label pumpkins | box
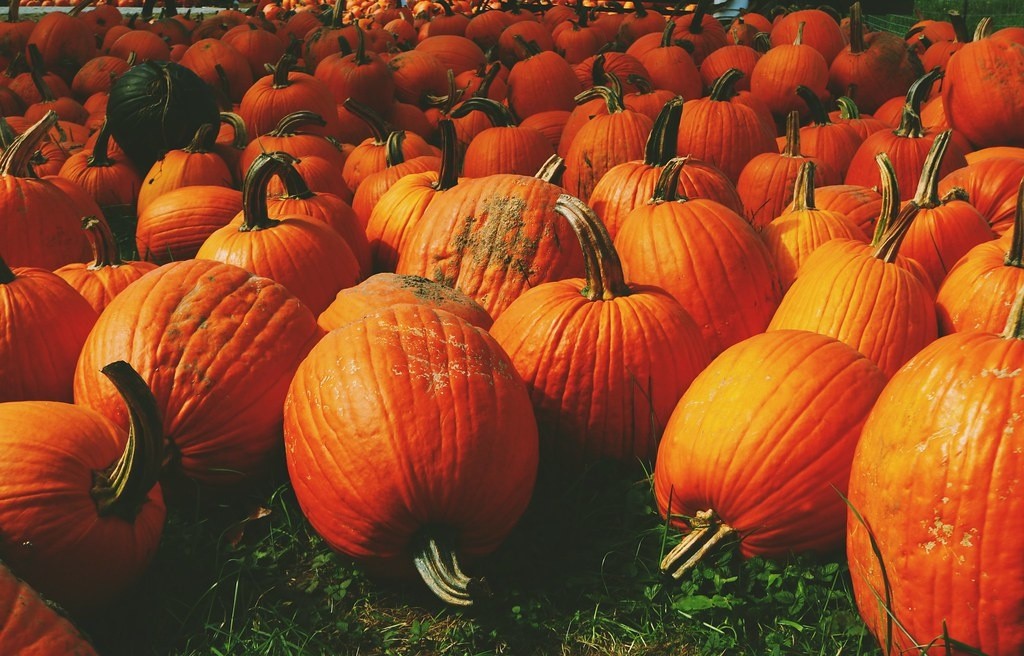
[0,0,1024,656]
